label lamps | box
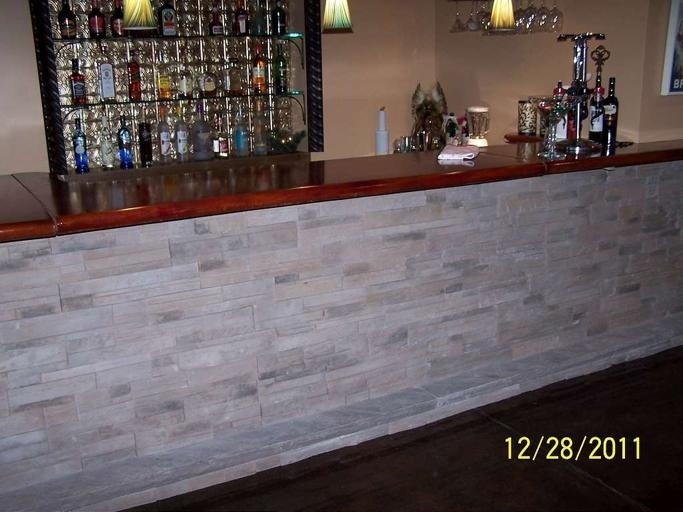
[121,0,158,31]
[321,0,354,34]
[489,0,516,31]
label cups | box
[465,105,491,146]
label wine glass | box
[526,96,579,160]
[449,0,563,33]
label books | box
[436,144,480,160]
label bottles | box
[554,80,565,99]
[58,1,291,38]
[71,108,292,171]
[67,36,288,107]
[589,75,618,146]
[375,106,389,156]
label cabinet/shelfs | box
[29,0,324,172]
